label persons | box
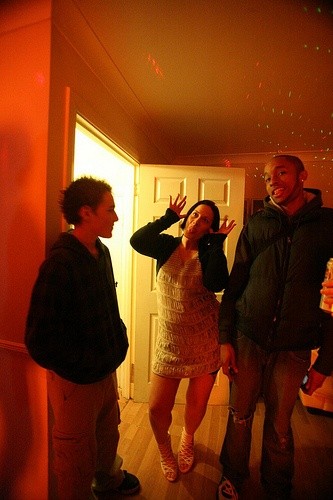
[320,280,333,305]
[24,175,140,500]
[216,155,333,500]
[130,193,237,482]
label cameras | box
[300,373,309,390]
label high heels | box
[177,426,195,473]
[157,431,178,482]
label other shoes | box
[92,470,140,495]
[217,475,239,500]
